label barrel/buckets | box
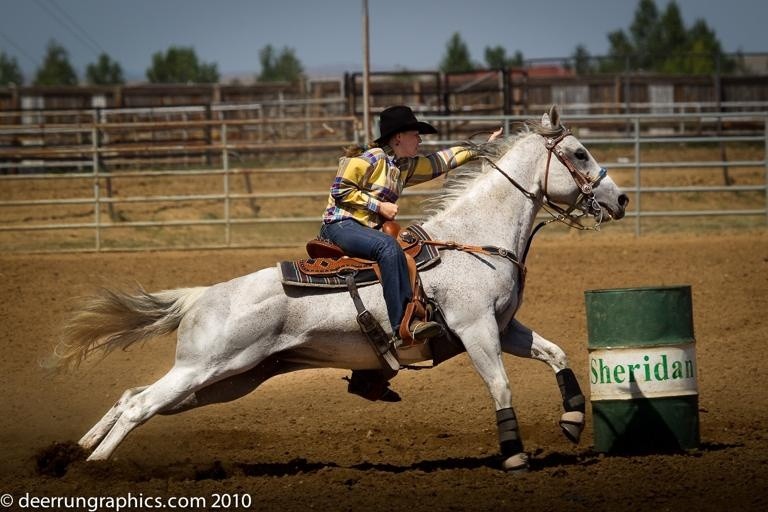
[584,285,700,454]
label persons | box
[320,127,503,350]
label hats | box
[374,106,437,143]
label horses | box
[35,103,630,476]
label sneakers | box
[394,316,442,351]
